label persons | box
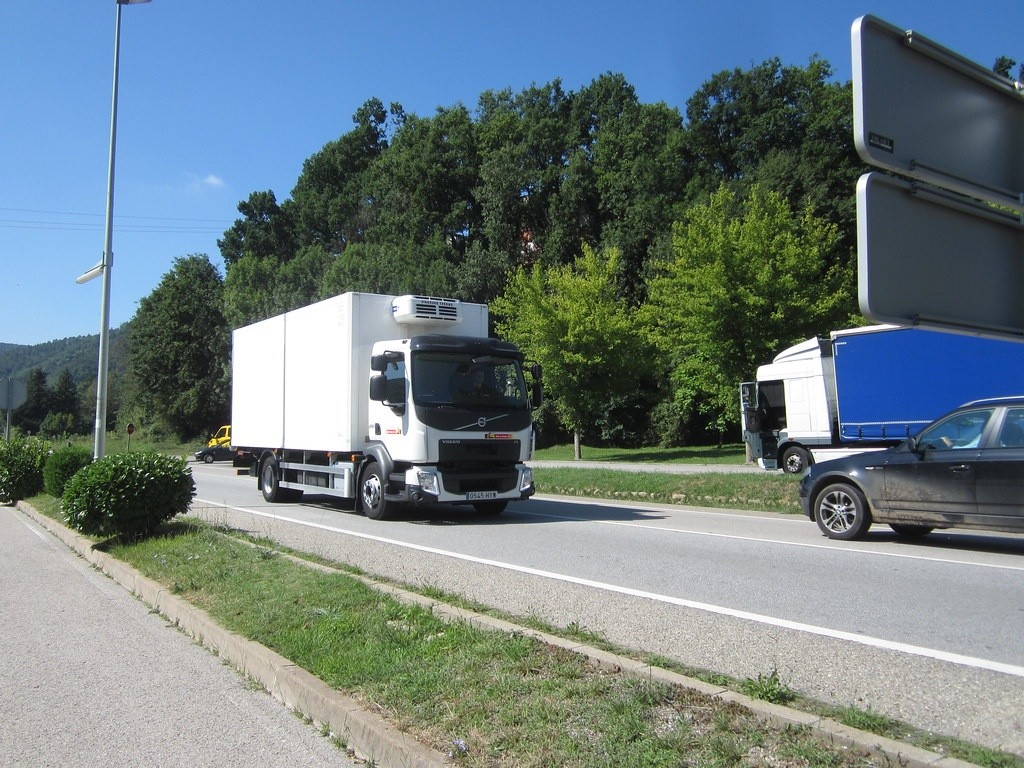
[461,369,502,397]
[940,419,1006,449]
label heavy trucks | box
[739,314,1024,475]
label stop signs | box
[127,424,134,435]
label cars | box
[195,439,234,463]
[799,391,1024,540]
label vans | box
[208,425,231,448]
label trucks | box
[230,289,544,519]
